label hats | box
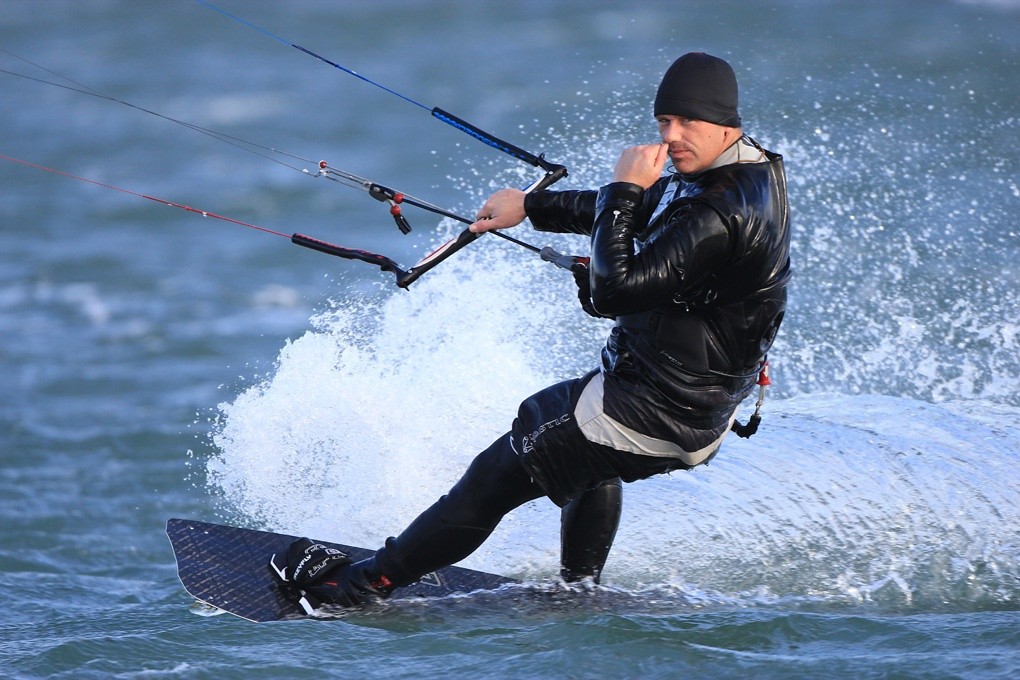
[654,51,742,128]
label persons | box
[288,52,793,609]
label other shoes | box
[287,538,389,617]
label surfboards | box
[165,516,526,627]
